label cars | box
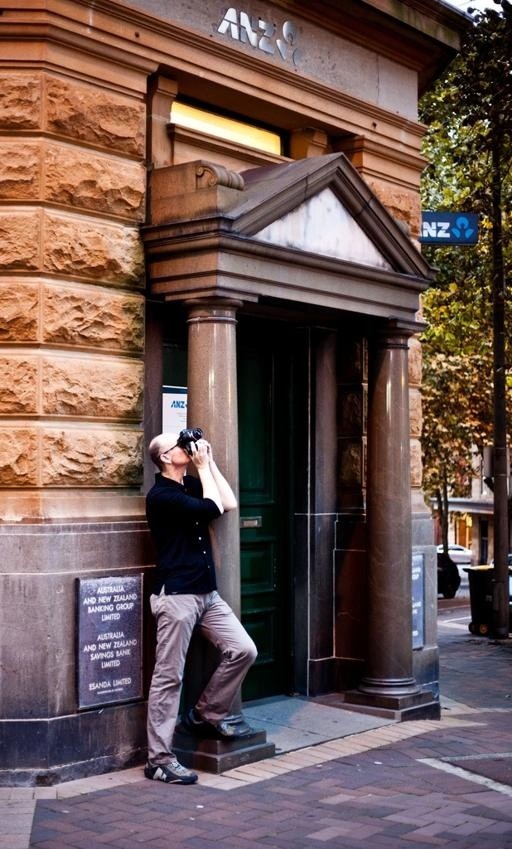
[435,544,472,565]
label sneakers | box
[189,708,253,739]
[143,758,199,786]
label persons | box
[141,430,259,786]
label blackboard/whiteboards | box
[74,573,144,710]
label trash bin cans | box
[462,564,512,639]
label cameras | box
[178,427,204,454]
